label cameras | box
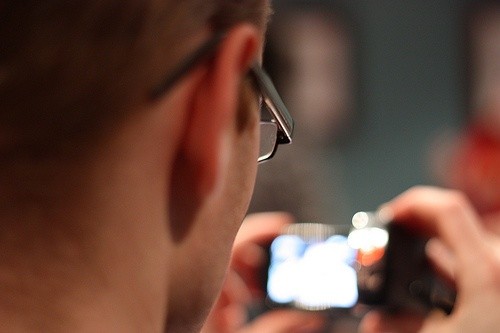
[264,221,457,316]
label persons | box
[0,0,500,332]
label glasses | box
[149,39,293,161]
